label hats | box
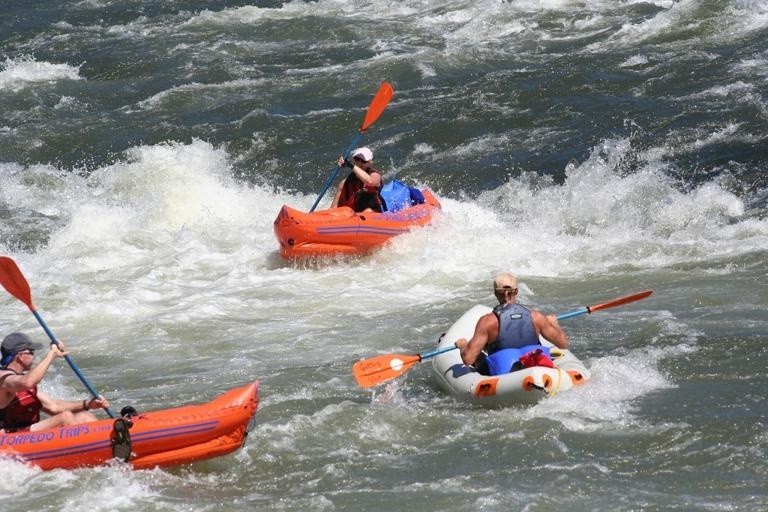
[352,148,373,161]
[494,273,517,292]
[1,333,44,356]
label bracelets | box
[83,400,88,410]
[348,161,355,169]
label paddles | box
[309,82,395,213]
[352,289,652,388]
[0,256,117,418]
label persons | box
[0,333,109,435]
[455,275,569,375]
[331,147,384,213]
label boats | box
[430,303,592,407]
[271,183,443,264]
[0,379,263,472]
